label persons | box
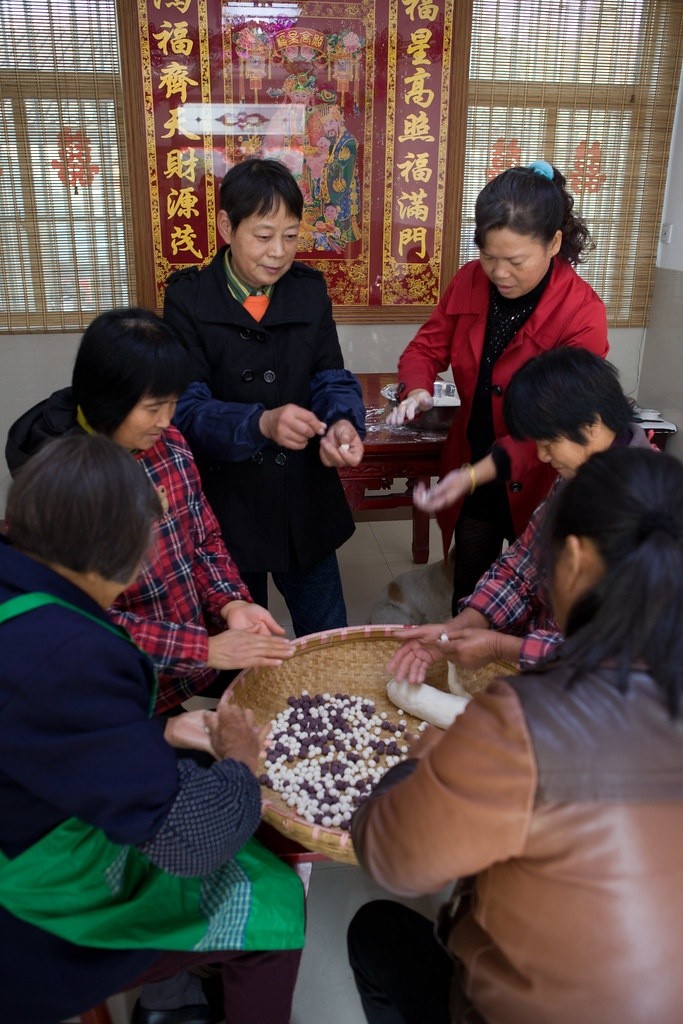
[385,161,611,618]
[0,432,308,1024]
[347,446,683,1024]
[163,159,366,641]
[5,307,337,862]
[383,334,662,687]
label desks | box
[334,372,460,564]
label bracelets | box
[461,463,478,500]
